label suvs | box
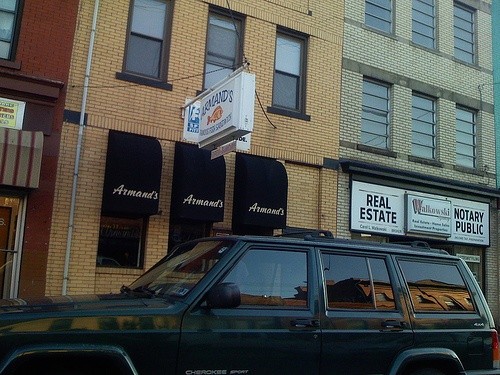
[0,229,500,375]
